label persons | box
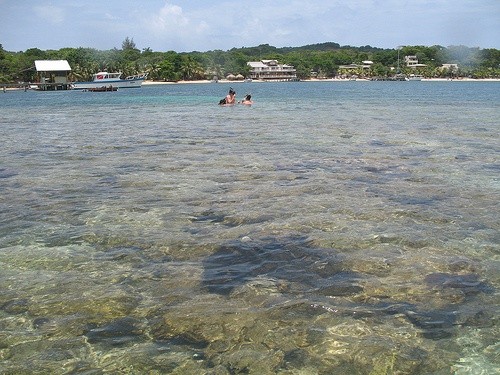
[218,98,226,106]
[226,90,237,105]
[240,95,254,104]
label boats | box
[63,72,149,88]
